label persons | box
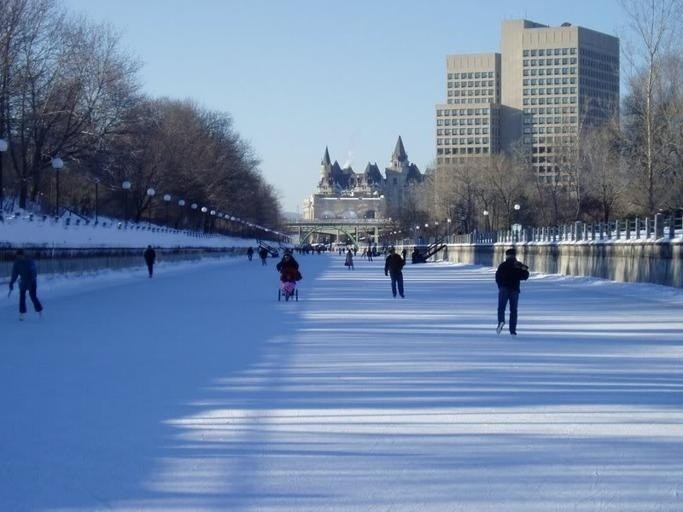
[244,242,419,298]
[9,250,42,322]
[494,247,530,336]
[143,245,155,278]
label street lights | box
[146,188,155,226]
[50,157,62,218]
[0,138,9,218]
[120,180,132,228]
[163,193,171,227]
[446,218,451,243]
[482,210,489,243]
[380,219,439,244]
[178,198,291,244]
[513,203,521,242]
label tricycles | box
[277,269,299,303]
